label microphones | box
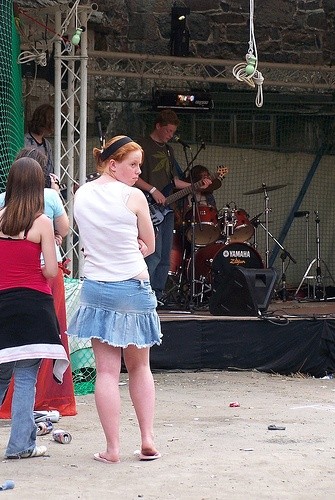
[174,135,190,148]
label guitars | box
[146,164,228,225]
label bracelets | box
[149,187,156,194]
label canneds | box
[229,402,240,407]
[34,410,60,435]
[52,430,72,443]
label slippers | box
[93,452,120,464]
[140,450,161,460]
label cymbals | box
[249,212,263,223]
[244,185,286,195]
[252,220,274,225]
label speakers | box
[209,265,280,316]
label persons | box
[0,158,68,458]
[0,147,77,418]
[21,104,53,157]
[130,109,212,308]
[180,165,216,215]
[64,136,163,464]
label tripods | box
[157,149,335,313]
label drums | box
[184,203,220,246]
[165,229,188,276]
[185,240,265,297]
[227,208,254,245]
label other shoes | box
[155,297,168,306]
[7,446,48,459]
